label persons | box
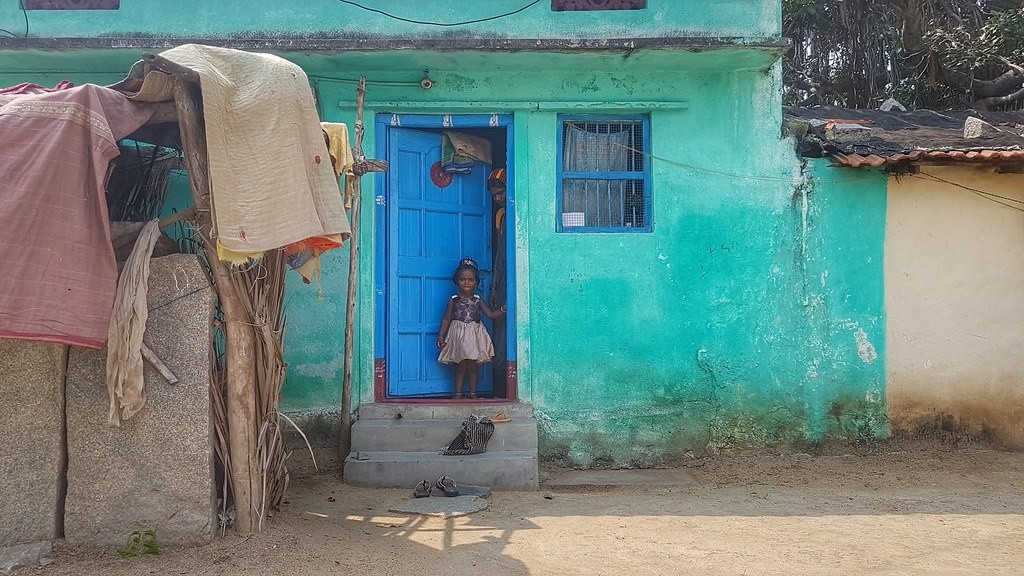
[487,167,506,398]
[438,258,506,399]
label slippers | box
[413,479,432,498]
[436,475,459,496]
[490,412,512,423]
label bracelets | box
[437,337,444,338]
[500,306,506,313]
[490,288,497,290]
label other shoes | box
[452,393,463,399]
[467,393,477,400]
